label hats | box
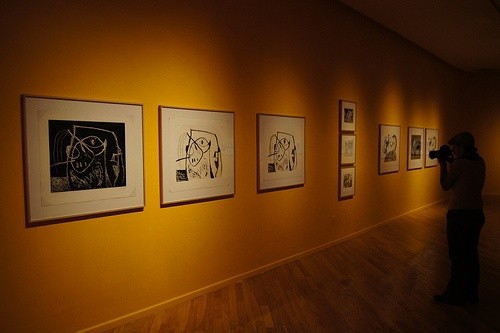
[448,132,475,147]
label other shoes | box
[433,290,480,311]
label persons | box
[433,131,486,310]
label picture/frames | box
[21,94,145,223]
[340,167,355,197]
[256,113,305,194]
[407,127,423,171]
[425,128,439,168]
[158,105,234,208]
[341,134,356,165]
[379,124,400,175]
[340,99,356,133]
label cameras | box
[429,145,454,163]
